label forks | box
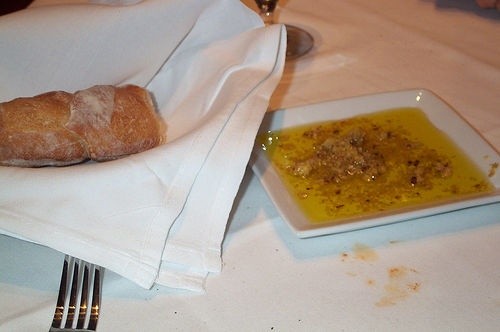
[48,255,102,332]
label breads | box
[0,85,164,167]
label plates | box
[250,88,500,239]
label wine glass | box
[254,0,313,59]
